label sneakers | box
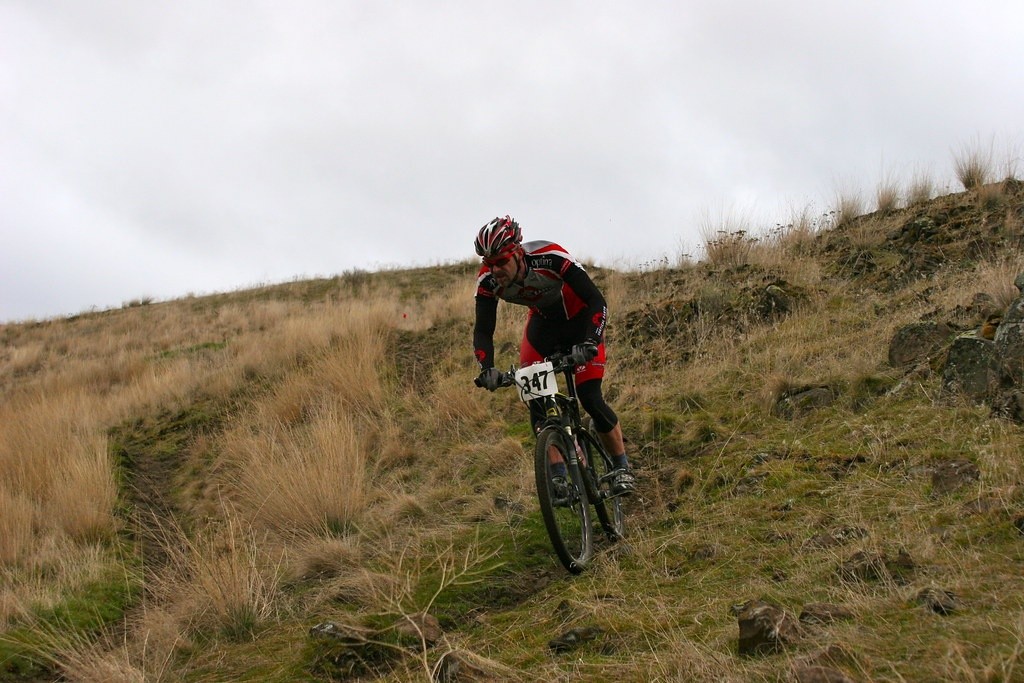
[552,477,572,508]
[610,465,635,497]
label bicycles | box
[474,346,633,575]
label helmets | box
[474,216,522,258]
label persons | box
[472,215,635,506]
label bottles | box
[573,440,587,468]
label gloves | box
[478,369,503,393]
[572,340,598,366]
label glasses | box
[482,248,519,267]
